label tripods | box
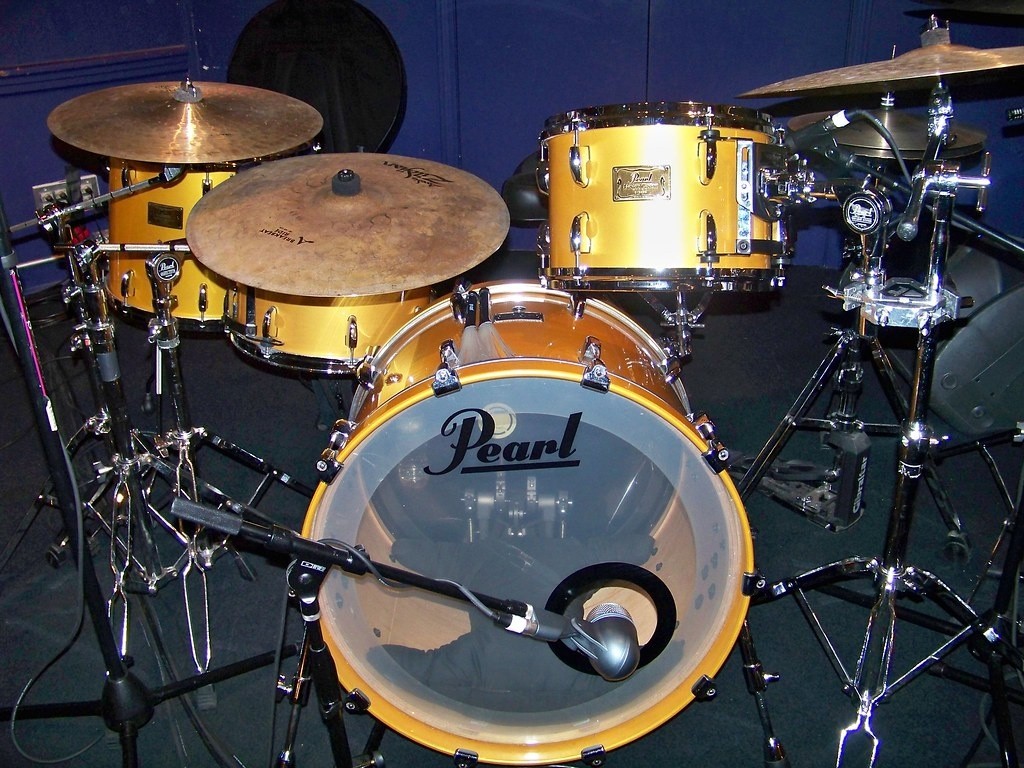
[731,85,1024,768]
[0,163,320,768]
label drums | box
[101,152,234,335]
[291,280,756,768]
[221,281,443,376]
[535,101,802,298]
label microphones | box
[583,603,640,681]
[783,107,859,154]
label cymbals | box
[790,105,988,165]
[738,44,1024,99]
[47,78,327,167]
[183,152,512,298]
